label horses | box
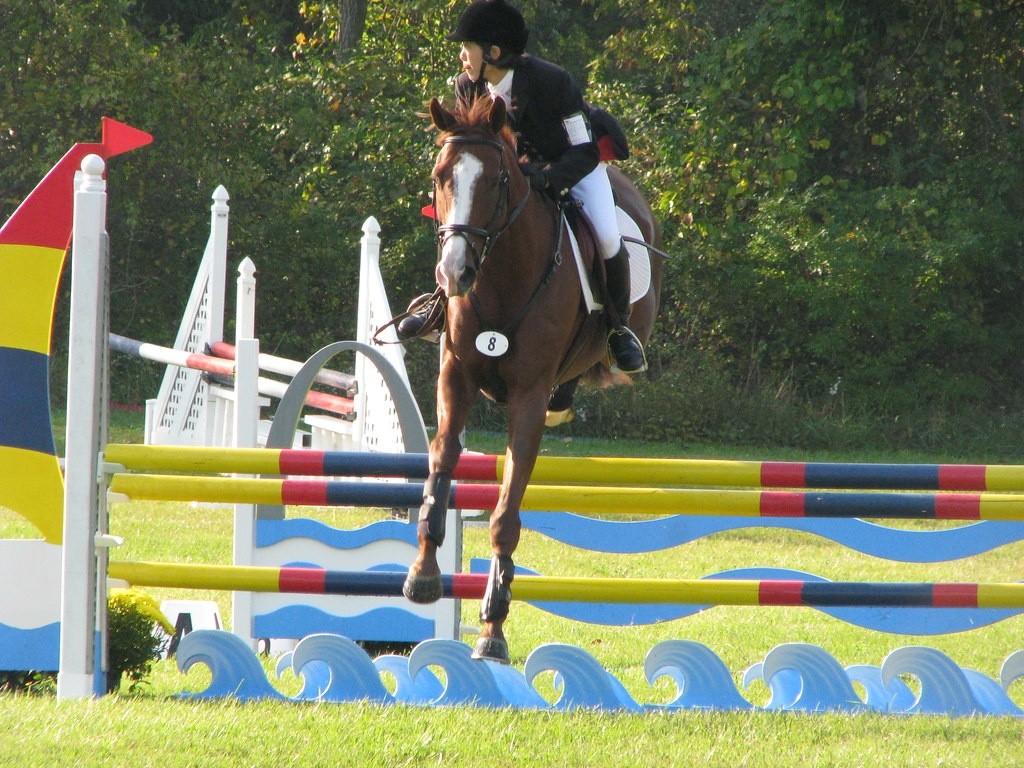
[401,90,664,665]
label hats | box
[444,0,530,52]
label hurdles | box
[0,140,1023,715]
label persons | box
[397,1,644,369]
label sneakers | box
[607,320,646,372]
[398,298,445,335]
